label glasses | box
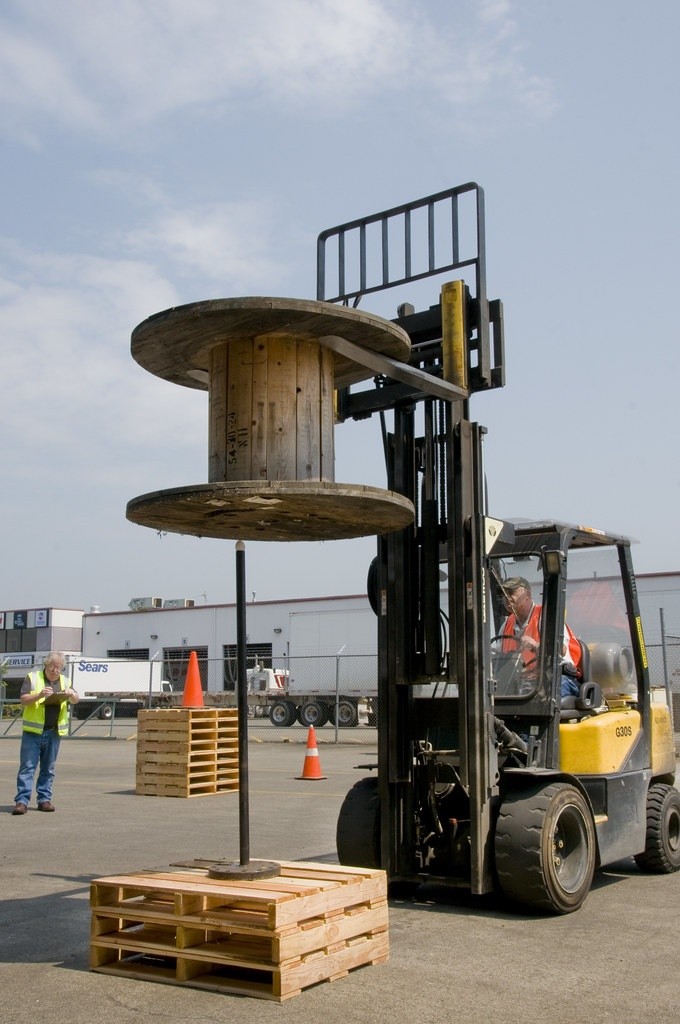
[47,664,63,671]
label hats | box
[496,576,531,591]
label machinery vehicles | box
[188,181,680,916]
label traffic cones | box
[293,725,328,780]
[173,651,210,710]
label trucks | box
[65,655,173,720]
[85,656,378,726]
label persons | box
[490,576,584,697]
[12,651,79,815]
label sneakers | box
[38,801,55,811]
[13,803,28,815]
